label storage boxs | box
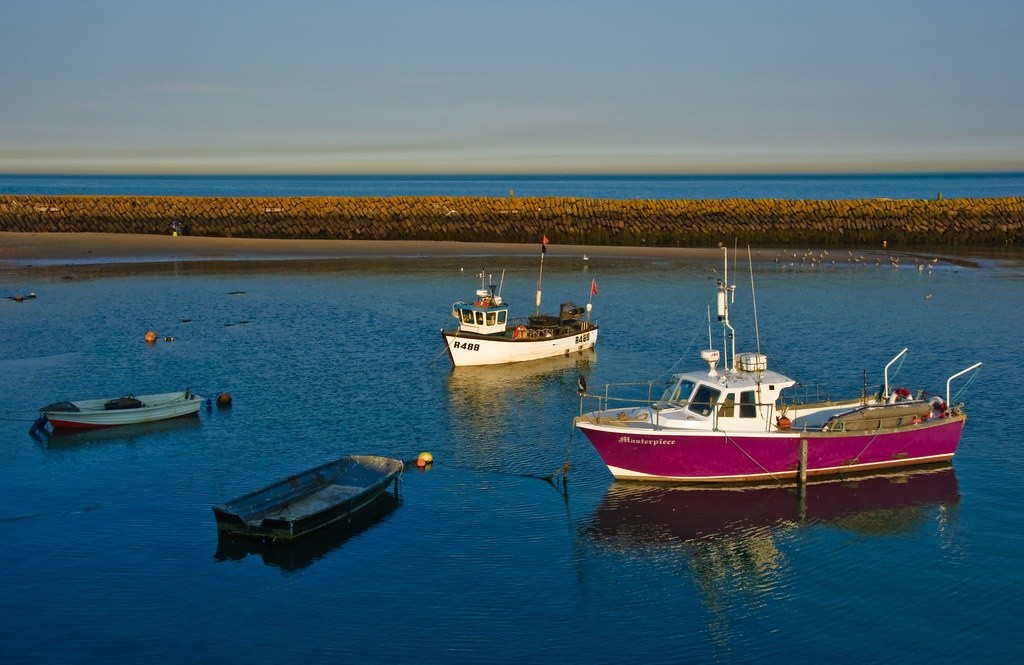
[734,352,769,370]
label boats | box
[28,385,206,436]
[211,456,403,540]
[440,234,599,367]
[573,238,982,484]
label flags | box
[593,282,598,294]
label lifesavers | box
[512,325,528,338]
[887,388,913,405]
[926,396,947,421]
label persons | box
[690,388,702,414]
